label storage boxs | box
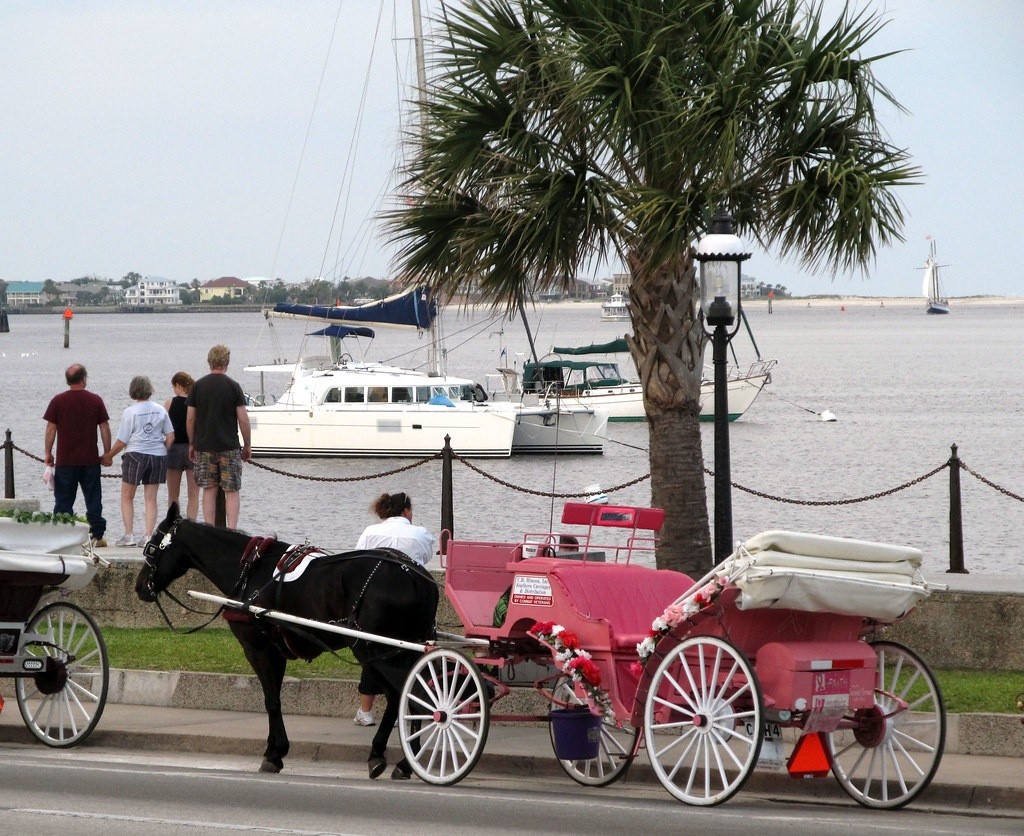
[755,639,878,712]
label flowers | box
[634,574,730,662]
[529,621,615,721]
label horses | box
[135,500,440,781]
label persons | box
[185,344,251,530]
[42,363,113,547]
[165,372,196,523]
[544,533,582,555]
[353,492,433,726]
[99,375,175,546]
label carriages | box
[136,385,947,810]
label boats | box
[600,292,632,323]
[484,304,778,422]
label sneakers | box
[353,707,375,726]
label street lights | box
[691,202,754,570]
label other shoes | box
[115,533,136,546]
[95,539,107,547]
[138,534,152,548]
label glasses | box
[400,492,408,503]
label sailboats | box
[239,0,608,459]
[914,236,952,315]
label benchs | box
[506,535,663,577]
[551,566,696,649]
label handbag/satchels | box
[493,585,511,627]
